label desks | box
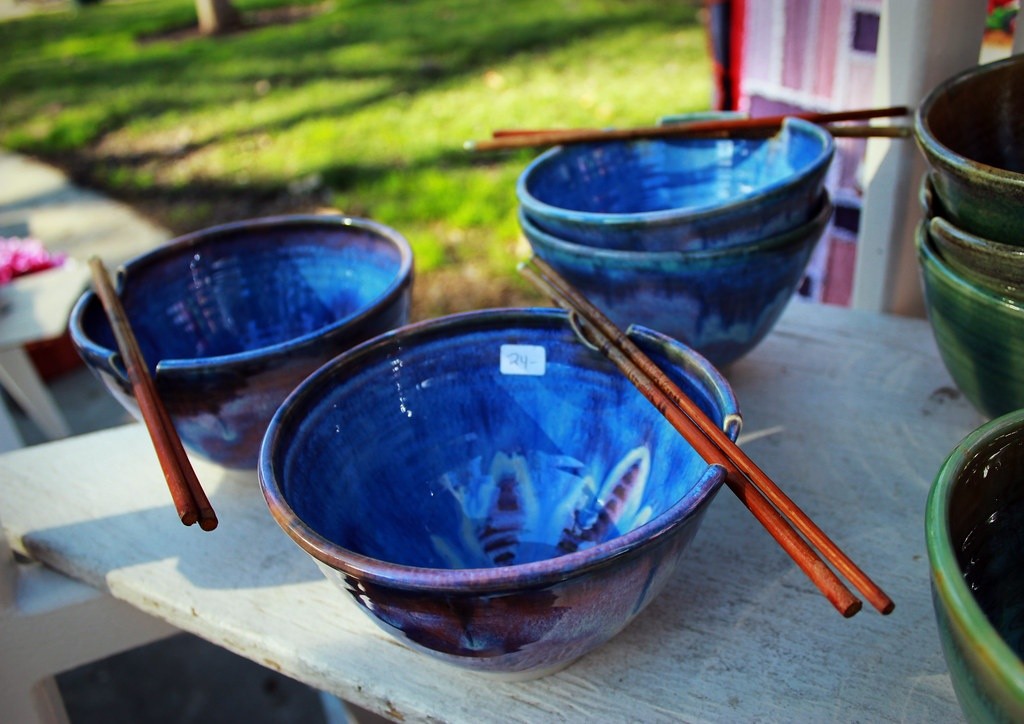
[1,292,994,724]
[1,150,180,440]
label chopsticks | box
[522,256,896,619]
[90,255,219,533]
[465,106,911,153]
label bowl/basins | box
[918,171,1024,298]
[259,307,741,683]
[518,112,835,251]
[70,213,415,471]
[924,410,1024,724]
[520,184,832,375]
[915,218,1024,419]
[913,53,1024,247]
